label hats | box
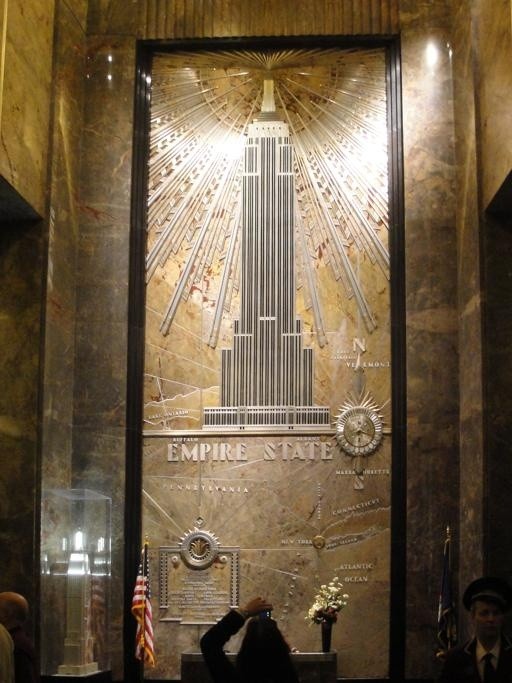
[462,577,512,607]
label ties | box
[480,653,497,683]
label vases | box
[320,621,333,652]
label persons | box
[0,592,29,683]
[438,576,512,683]
[200,597,298,683]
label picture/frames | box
[118,29,409,683]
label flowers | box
[303,576,348,627]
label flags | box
[132,539,155,668]
[86,545,106,664]
[436,538,458,662]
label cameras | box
[259,610,271,620]
